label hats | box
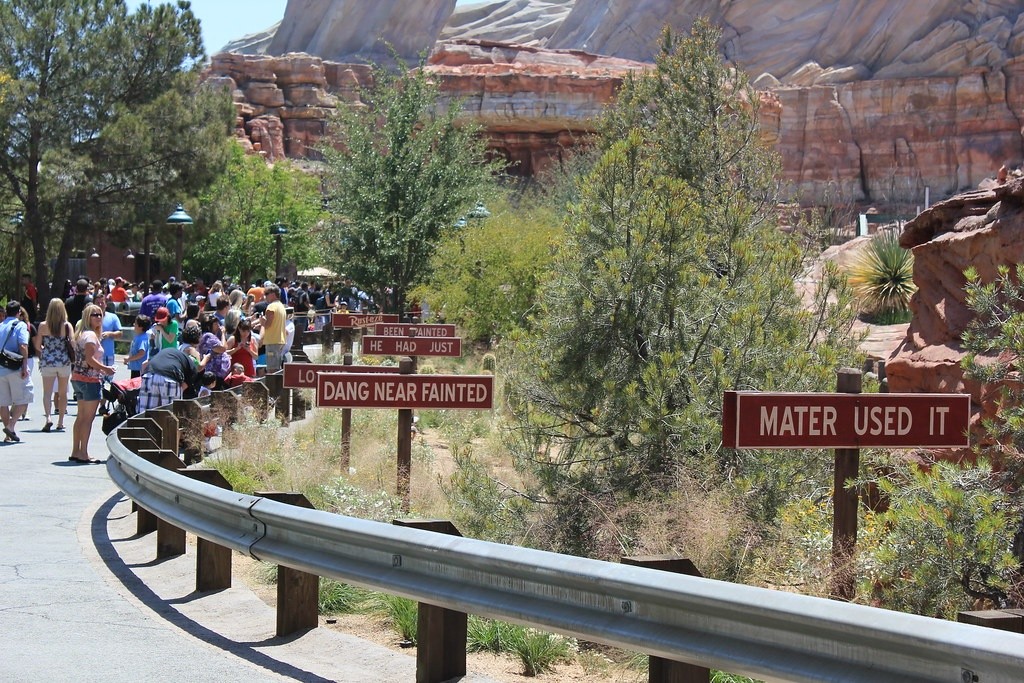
[341,301,347,305]
[155,306,170,322]
[153,280,163,289]
[77,279,88,291]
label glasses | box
[90,314,102,317]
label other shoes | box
[22,415,30,420]
[42,423,53,432]
[55,409,68,414]
[3,428,20,442]
[99,405,106,415]
[68,456,101,464]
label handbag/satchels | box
[65,321,75,363]
[307,309,315,318]
[0,349,24,370]
[102,411,127,436]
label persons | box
[148,307,178,360]
[123,314,152,379]
[196,313,232,392]
[68,305,116,464]
[1,275,435,464]
[260,283,287,374]
[36,298,76,432]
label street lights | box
[166,203,194,284]
[466,200,492,228]
[10,211,28,303]
[453,216,473,255]
[269,226,288,278]
